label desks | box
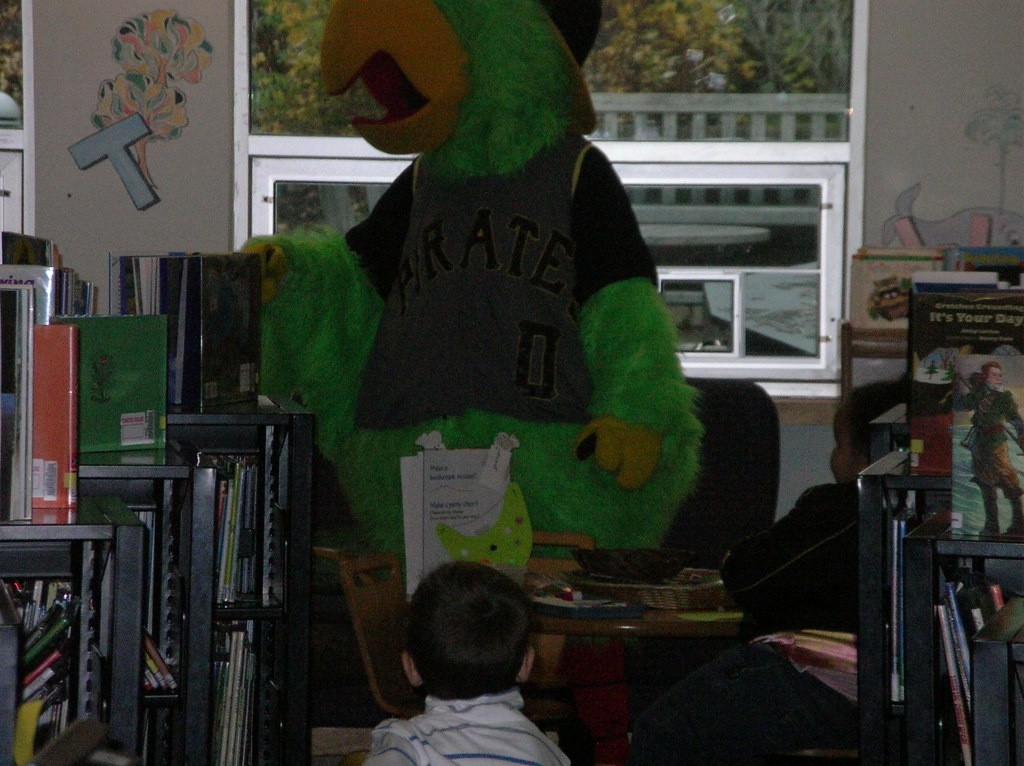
[525,606,741,766]
[640,224,770,266]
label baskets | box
[563,570,724,612]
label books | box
[0,228,277,766]
[846,242,1024,766]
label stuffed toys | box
[237,0,707,552]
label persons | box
[360,562,573,766]
[724,375,911,645]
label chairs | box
[525,532,594,682]
[340,552,426,716]
[658,374,782,550]
